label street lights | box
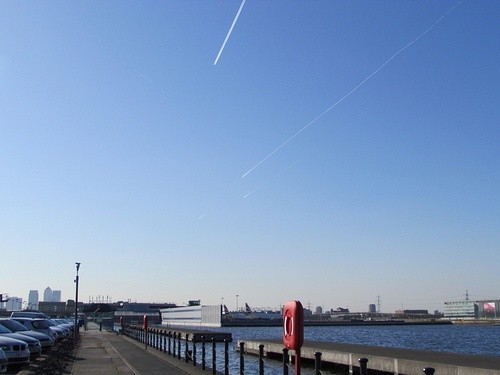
[74,262,81,334]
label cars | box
[0,311,79,373]
